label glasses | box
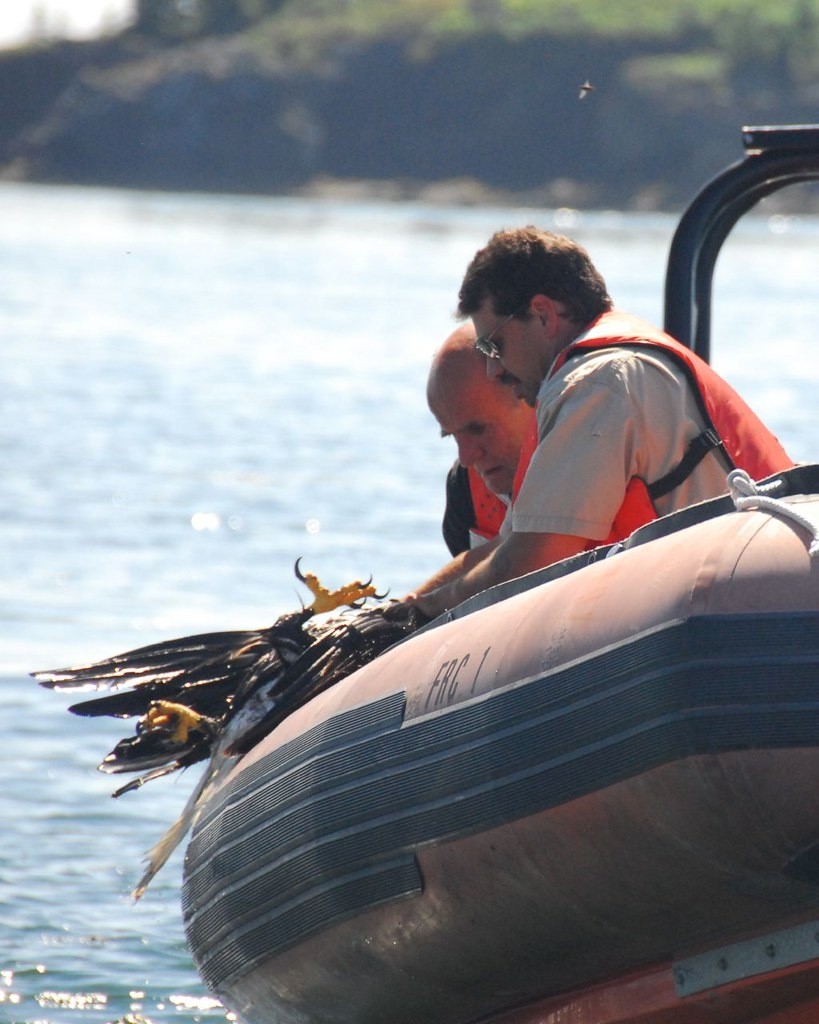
[474,305,529,358]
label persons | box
[404,224,794,620]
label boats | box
[174,122,819,1024]
